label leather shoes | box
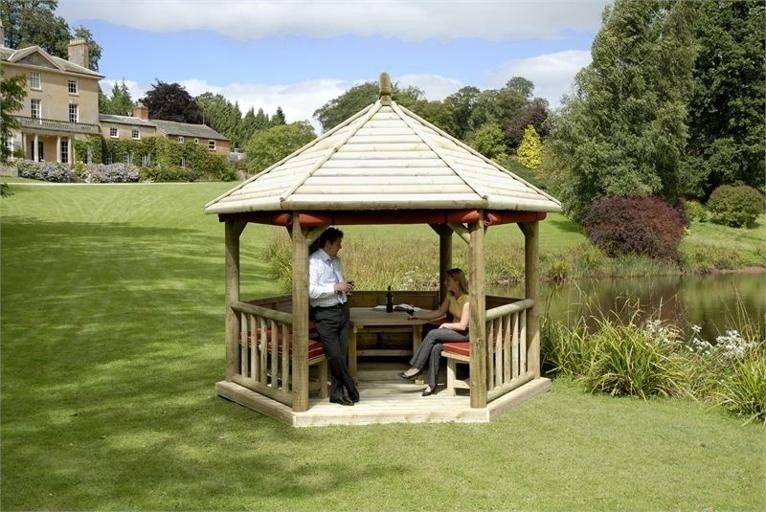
[329,395,353,406]
[343,378,359,402]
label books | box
[371,301,420,312]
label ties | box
[329,260,345,304]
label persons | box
[306,226,360,407]
[398,268,472,396]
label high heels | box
[400,367,422,379]
[422,384,437,396]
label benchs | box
[268,318,321,340]
[426,315,452,329]
[237,328,331,399]
[439,333,517,400]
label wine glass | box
[406,305,415,319]
[347,280,354,295]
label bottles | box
[385,284,394,312]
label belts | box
[315,303,344,310]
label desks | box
[339,307,447,389]
